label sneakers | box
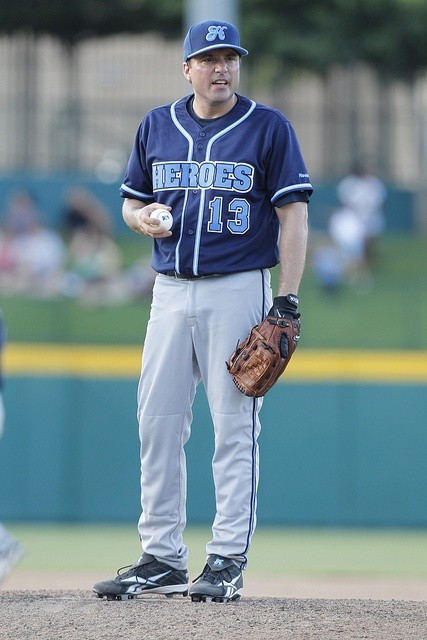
[187,554,244,602]
[92,553,189,603]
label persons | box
[93,20,312,599]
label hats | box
[183,20,249,60]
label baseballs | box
[149,209,174,231]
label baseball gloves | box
[224,293,302,398]
